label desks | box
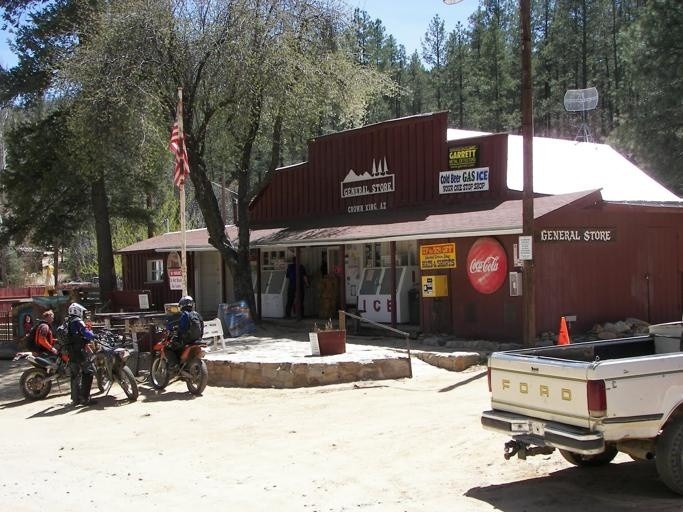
[88,310,168,345]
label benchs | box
[199,317,226,352]
[105,288,157,313]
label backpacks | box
[183,311,202,340]
[26,323,42,352]
[56,317,77,346]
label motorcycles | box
[11,338,96,401]
[93,329,138,401]
[148,323,208,395]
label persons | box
[62,302,99,406]
[169,294,206,371]
[284,255,309,317]
[32,311,66,377]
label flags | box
[165,100,191,191]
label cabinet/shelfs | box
[345,243,384,305]
[258,246,298,270]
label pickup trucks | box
[479,318,683,496]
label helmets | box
[68,303,87,318]
[178,295,194,311]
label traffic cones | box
[557,315,572,345]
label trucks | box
[62,276,98,287]
